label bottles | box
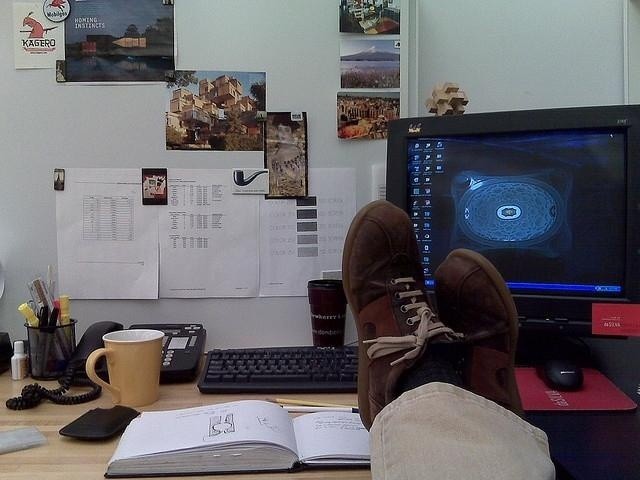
[10,341,27,381]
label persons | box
[340,198,560,480]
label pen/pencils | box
[266,398,359,412]
[18,264,71,374]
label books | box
[103,397,372,480]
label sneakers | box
[338,195,457,429]
[432,247,525,424]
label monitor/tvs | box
[385,104,639,368]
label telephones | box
[73,321,206,384]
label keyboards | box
[197,345,360,394]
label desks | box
[2,338,639,480]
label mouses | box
[542,360,583,390]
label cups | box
[85,328,167,409]
[308,279,348,348]
